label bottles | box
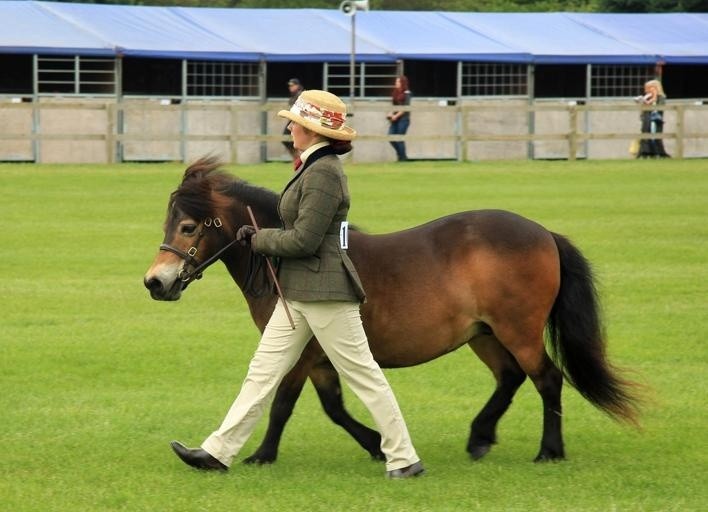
[278,89,356,142]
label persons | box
[170,88,422,486]
[281,78,310,171]
[635,78,670,158]
[385,75,412,161]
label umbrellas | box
[170,440,227,472]
[386,459,425,480]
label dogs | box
[144,150,652,465]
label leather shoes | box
[236,224,261,247]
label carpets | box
[294,157,302,171]
